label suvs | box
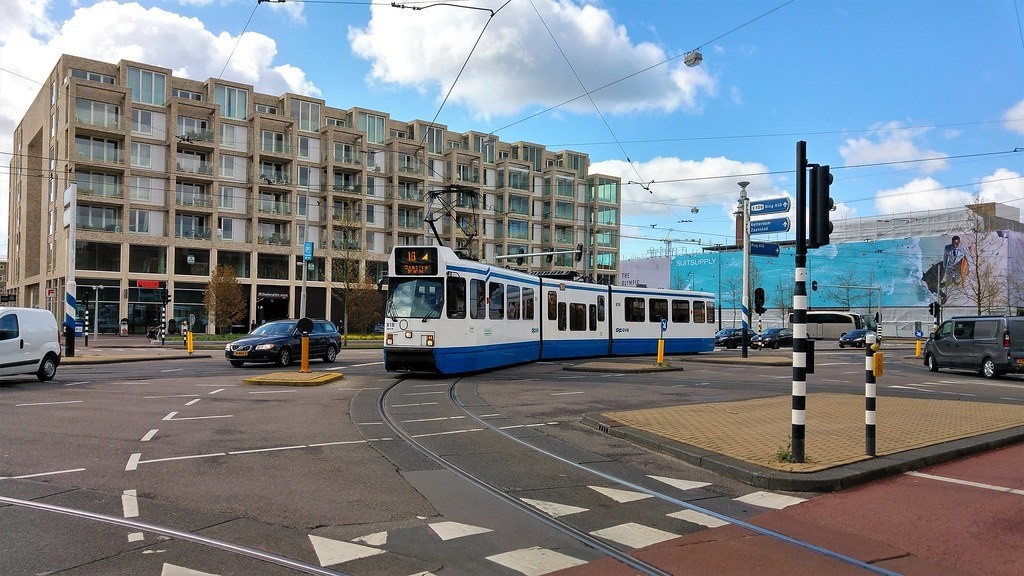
[224,318,342,368]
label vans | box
[0,306,62,381]
[922,314,1024,379]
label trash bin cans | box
[168,319,177,335]
[120,318,129,336]
[75,320,85,336]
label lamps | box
[372,165,382,174]
[684,50,704,67]
[691,206,699,214]
[483,134,496,145]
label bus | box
[786,310,878,341]
[373,184,716,375]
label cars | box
[715,327,757,349]
[749,328,793,349]
[838,330,881,349]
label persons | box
[942,236,973,283]
[458,306,463,316]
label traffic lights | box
[161,288,172,306]
[928,302,941,315]
[809,164,834,247]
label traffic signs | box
[749,241,780,257]
[750,218,791,233]
[750,197,791,215]
